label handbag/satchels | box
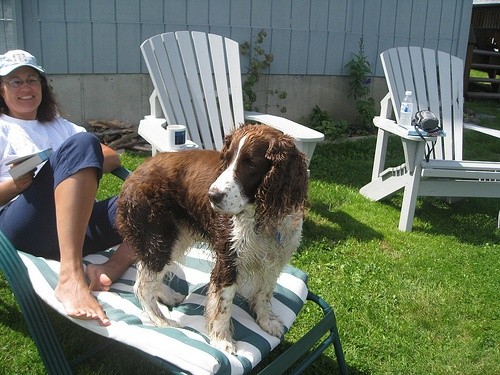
[412,110,441,162]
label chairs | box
[138,31,324,178]
[359,46,500,233]
[0,163,350,375]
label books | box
[4,147,55,182]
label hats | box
[0,50,44,76]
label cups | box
[166,124,187,150]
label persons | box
[0,50,138,326]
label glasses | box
[0,77,40,89]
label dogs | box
[113,123,310,357]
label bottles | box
[398,90,414,126]
